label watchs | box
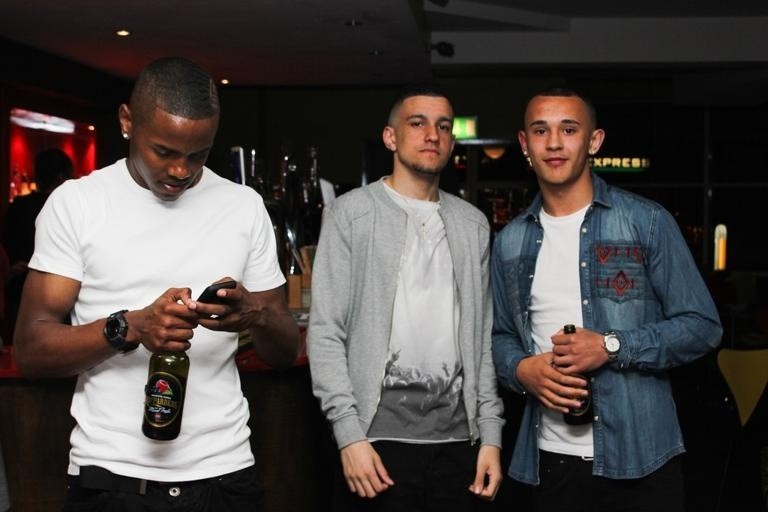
[104,309,141,354]
[602,329,621,361]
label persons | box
[8,148,76,267]
[489,81,726,510]
[10,55,303,511]
[303,83,509,512]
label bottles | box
[556,324,595,427]
[142,351,190,440]
